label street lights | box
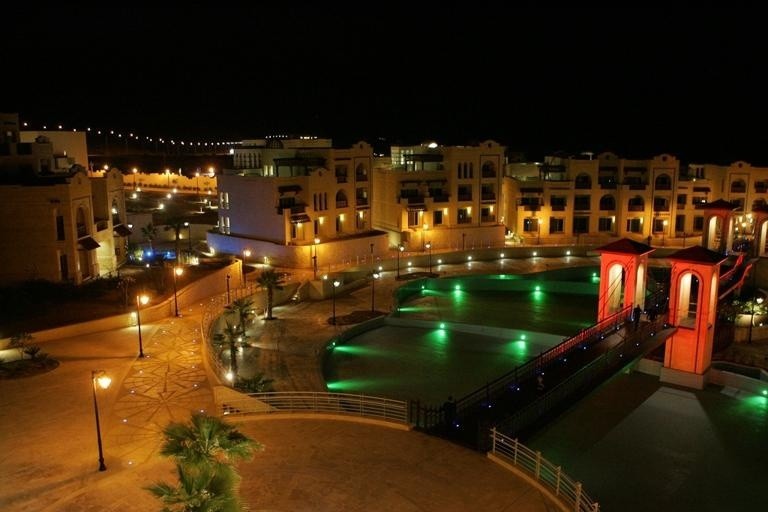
[661,218,668,246]
[182,221,193,255]
[174,265,185,318]
[241,246,253,287]
[748,292,765,342]
[535,216,542,246]
[395,243,405,278]
[425,241,433,275]
[135,291,148,358]
[313,236,320,277]
[370,270,380,315]
[91,369,111,471]
[127,220,134,256]
[328,274,341,323]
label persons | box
[646,303,658,322]
[633,303,642,331]
